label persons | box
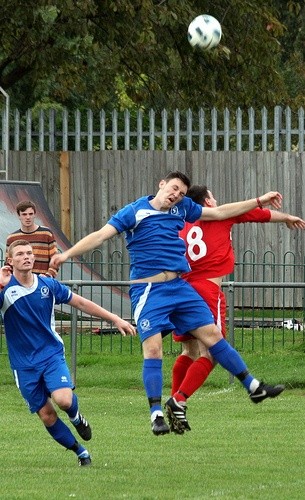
[0,239,136,466]
[4,201,59,279]
[50,171,285,436]
[164,184,305,434]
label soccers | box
[187,14,222,51]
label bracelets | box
[0,284,4,289]
[256,197,263,209]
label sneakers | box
[249,378,285,404]
[69,411,91,441]
[164,396,191,434]
[78,449,91,466]
[151,410,170,436]
